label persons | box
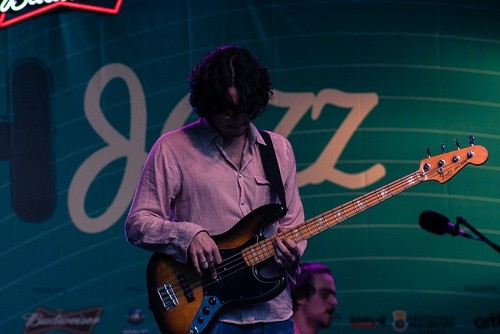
[125,46,307,334]
[291,262,338,333]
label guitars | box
[146,135,489,334]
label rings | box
[207,257,214,262]
[200,260,206,264]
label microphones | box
[418,210,473,239]
